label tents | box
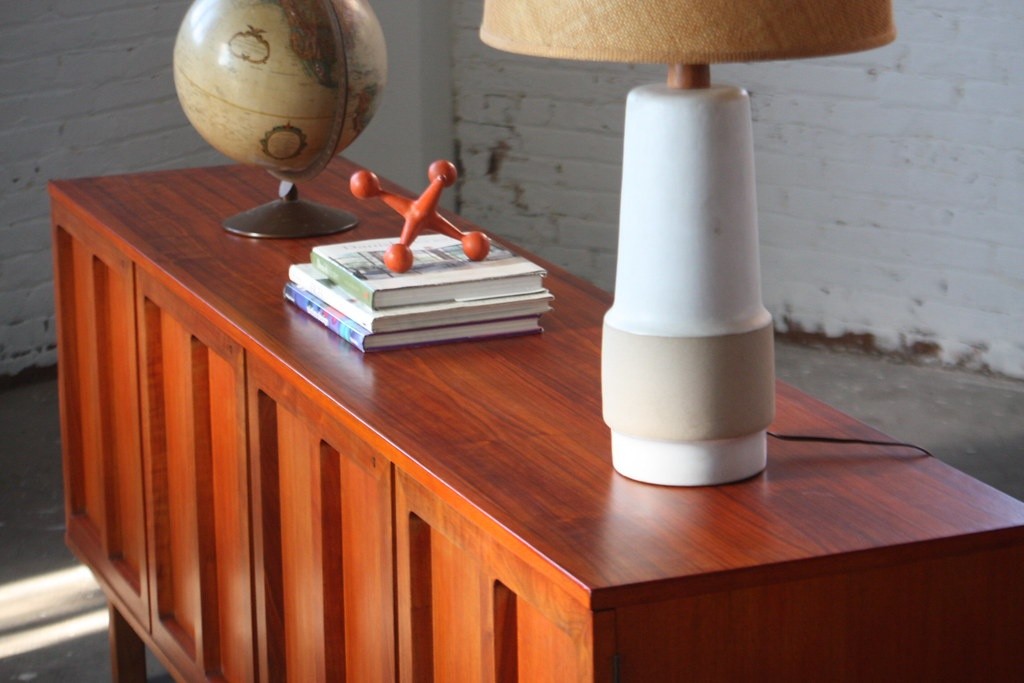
[282,263,556,354]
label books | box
[310,232,548,311]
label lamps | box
[477,0,899,493]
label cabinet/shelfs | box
[30,155,1024,683]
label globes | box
[170,0,390,240]
